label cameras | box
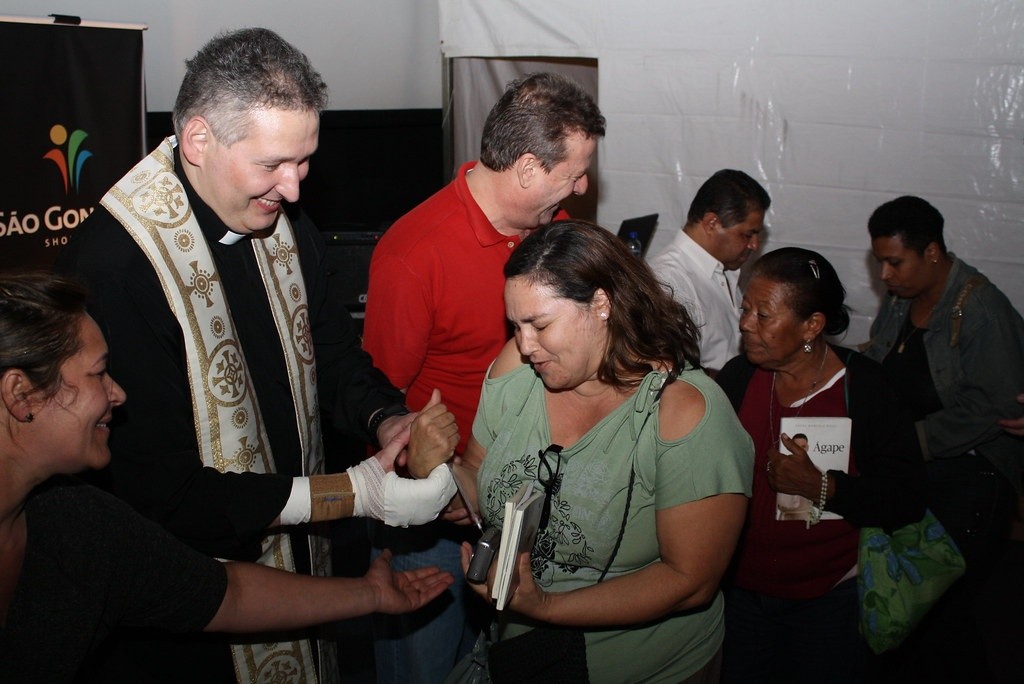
[468,526,502,584]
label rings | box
[766,461,772,471]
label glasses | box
[538,444,563,531]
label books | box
[775,417,852,521]
[491,484,546,611]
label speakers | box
[323,231,383,339]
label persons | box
[645,169,771,381]
[362,73,606,684]
[405,220,756,684]
[715,246,928,684]
[46,28,458,684]
[863,195,1024,684]
[778,434,816,520]
[0,267,453,684]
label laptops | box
[616,213,660,258]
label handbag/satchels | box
[856,509,966,656]
[488,623,591,684]
[442,617,499,684]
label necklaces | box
[769,344,828,448]
[897,304,935,354]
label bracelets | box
[811,474,828,524]
[369,403,409,445]
